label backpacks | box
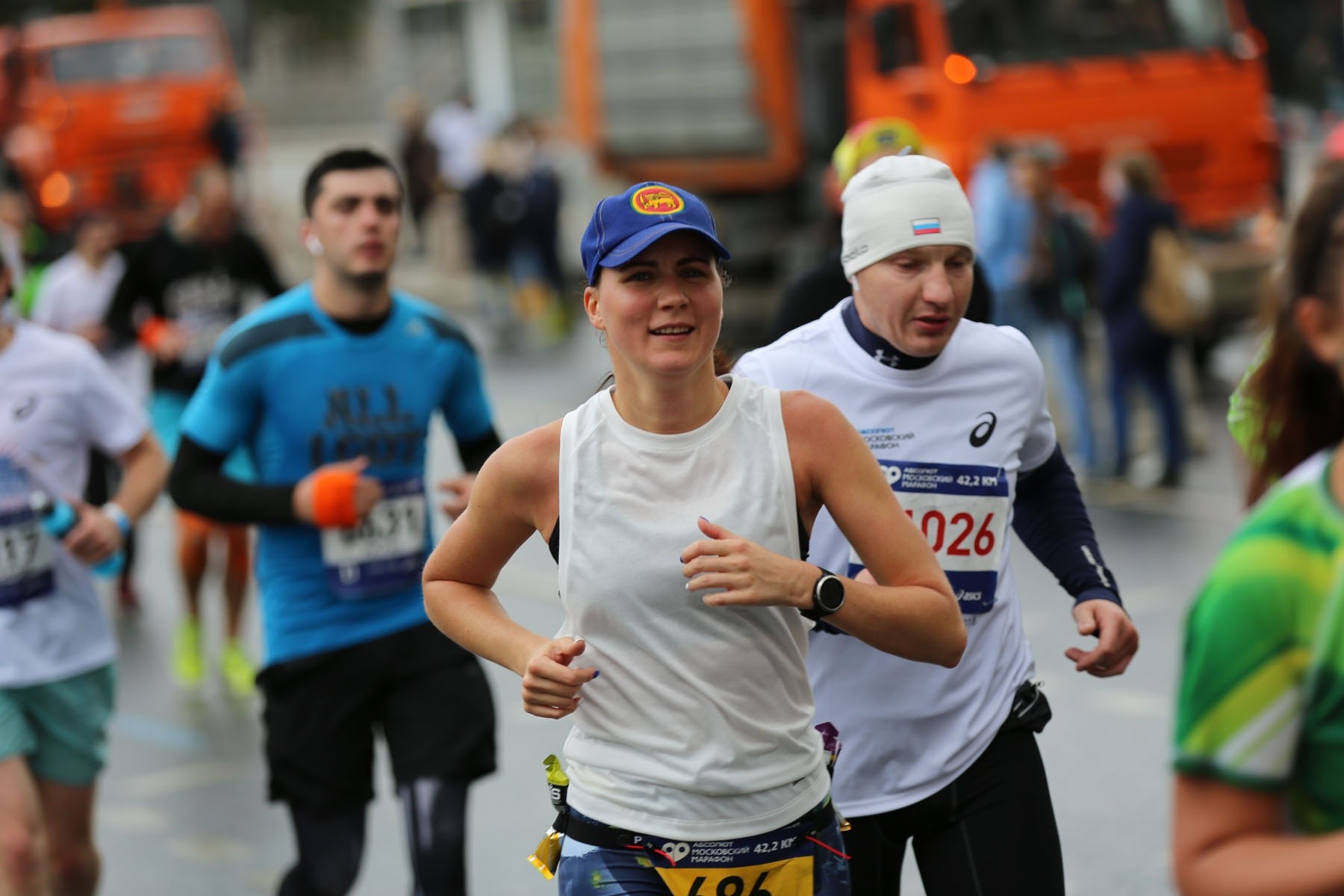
[1140,224,1214,332]
[1034,212,1099,320]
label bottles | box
[28,491,128,580]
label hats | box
[580,180,732,287]
[831,115,922,181]
[840,153,977,278]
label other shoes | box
[1110,449,1133,480]
[1158,440,1192,488]
[216,636,255,696]
[170,615,209,686]
[115,583,141,609]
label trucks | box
[0,7,244,243]
[557,1,1287,318]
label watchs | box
[798,563,843,619]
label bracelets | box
[100,499,133,538]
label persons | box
[0,96,290,702]
[1167,159,1344,896]
[725,154,1142,896]
[762,122,998,345]
[398,102,567,359]
[968,136,1110,494]
[1085,152,1190,494]
[167,149,506,896]
[418,180,968,896]
[0,162,172,896]
